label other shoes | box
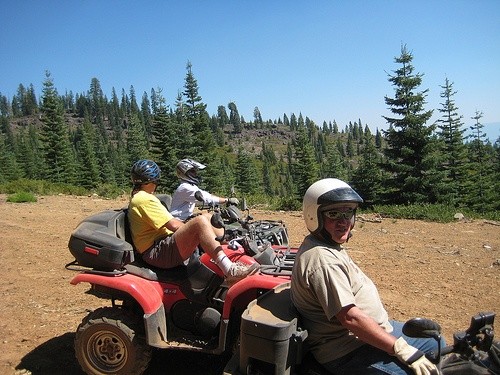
[226,263,260,288]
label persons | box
[291,178,447,375]
[127,160,259,290]
[168,159,239,239]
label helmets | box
[175,158,206,186]
[130,158,162,185]
[302,177,364,235]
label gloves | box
[228,198,239,205]
[392,336,440,375]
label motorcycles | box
[221,279,500,375]
[61,195,300,375]
